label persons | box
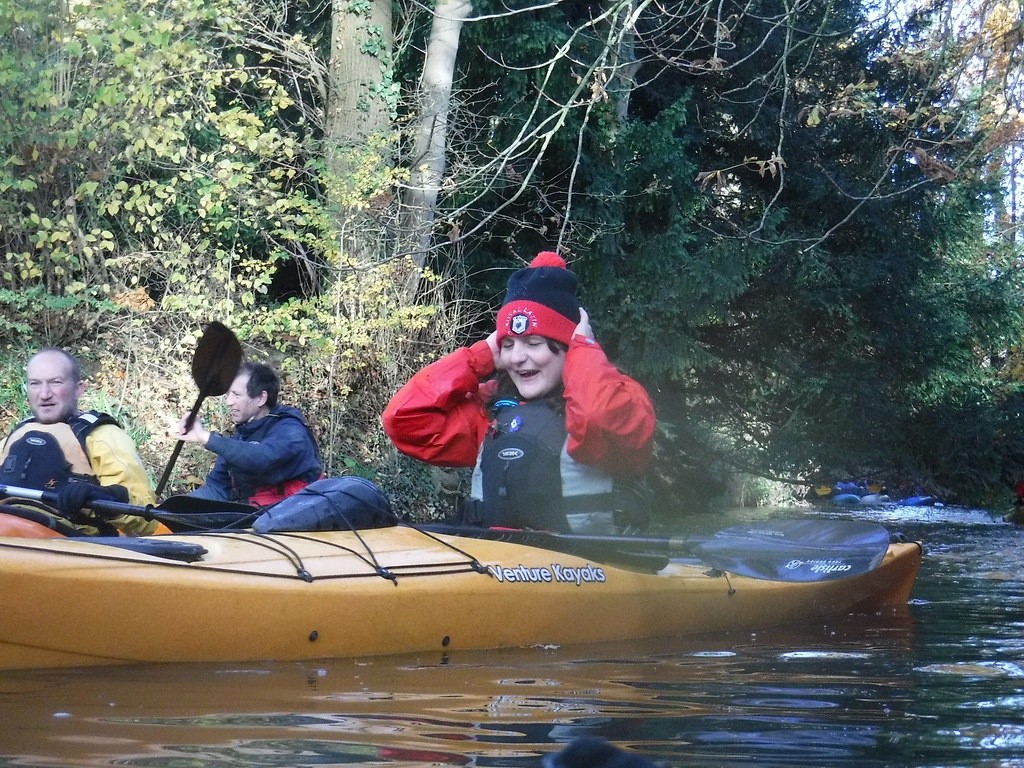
[175,362,325,508]
[0,347,159,536]
[382,251,657,536]
[804,471,887,504]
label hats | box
[494,251,582,354]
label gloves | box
[55,483,127,522]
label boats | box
[1,498,920,669]
[822,482,936,505]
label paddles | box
[0,485,263,534]
[153,320,243,504]
[413,519,890,584]
